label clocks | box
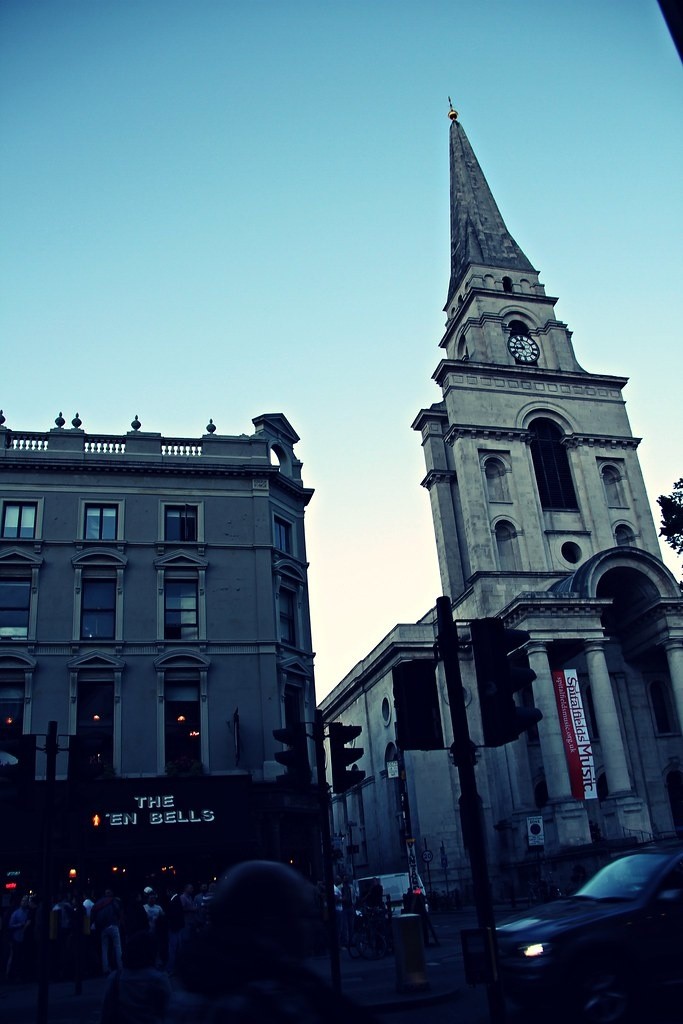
[506,333,541,364]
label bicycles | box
[349,908,389,961]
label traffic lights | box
[271,727,311,791]
[329,722,367,793]
[469,613,542,750]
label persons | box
[319,868,464,954]
[527,872,560,903]
[187,860,378,1024]
[1,878,216,980]
[99,929,168,1024]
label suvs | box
[493,835,683,1023]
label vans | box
[347,871,430,916]
